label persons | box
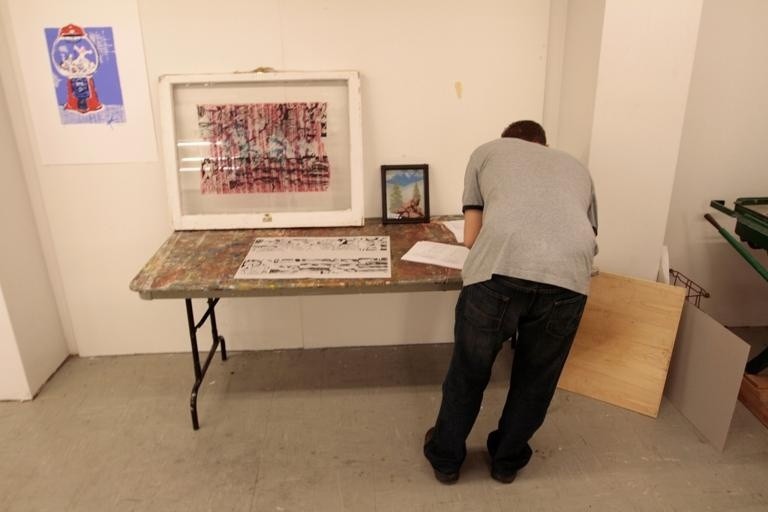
[422,118,598,485]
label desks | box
[130,214,519,431]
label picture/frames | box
[381,165,430,225]
[158,70,364,232]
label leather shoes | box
[423,426,467,485]
[487,429,533,483]
